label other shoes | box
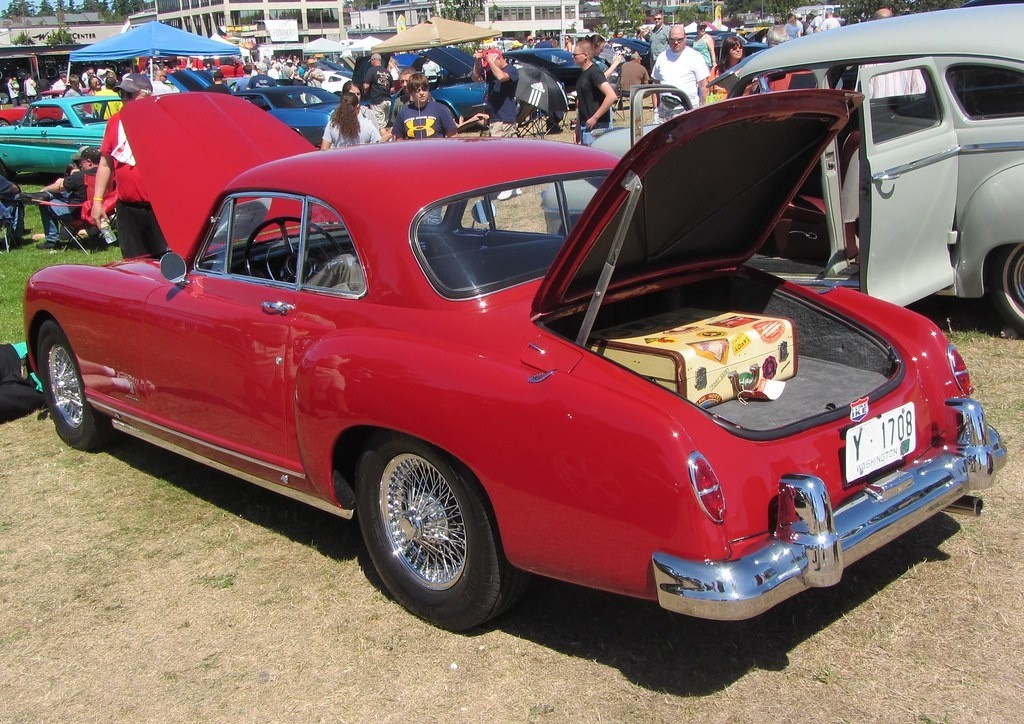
[23,228,31,236]
[649,106,658,112]
[3,237,24,250]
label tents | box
[304,37,345,53]
[371,16,500,54]
[66,21,243,84]
[347,36,385,52]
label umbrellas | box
[515,62,569,119]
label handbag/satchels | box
[708,66,721,92]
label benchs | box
[428,238,565,288]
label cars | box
[23,90,1007,632]
[167,57,368,148]
[0,90,126,186]
[418,28,770,125]
[540,4,1024,334]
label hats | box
[307,58,317,65]
[71,146,91,161]
[631,53,642,60]
[113,73,153,95]
[255,62,268,72]
[481,48,501,68]
[368,53,382,63]
[286,59,294,65]
[510,40,523,49]
[697,22,707,30]
[526,36,533,42]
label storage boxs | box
[591,307,799,411]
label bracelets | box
[93,198,103,201]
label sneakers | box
[36,241,62,252]
[19,191,50,207]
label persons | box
[0,10,926,247]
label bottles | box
[100,217,117,244]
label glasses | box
[731,45,742,51]
[413,86,428,93]
[352,92,361,96]
[399,80,408,85]
[572,52,587,57]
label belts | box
[120,200,151,210]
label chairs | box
[515,107,544,140]
[609,75,626,122]
[0,201,18,253]
[50,198,116,255]
